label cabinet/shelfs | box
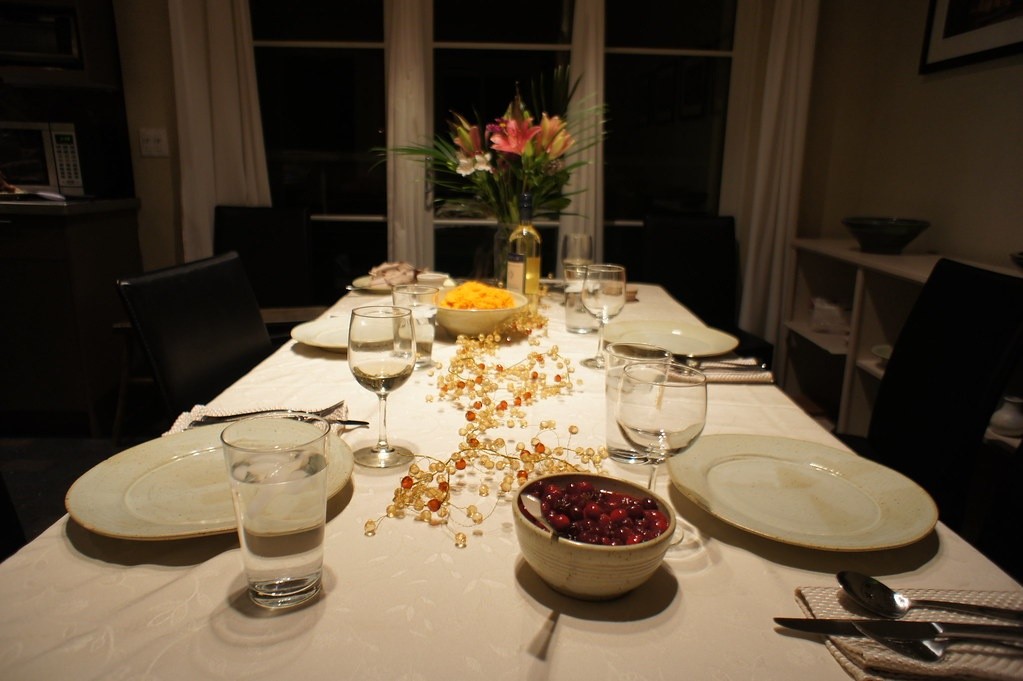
[778,235,1023,583]
[0,194,141,439]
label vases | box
[493,222,520,286]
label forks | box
[201,399,344,416]
[848,619,1023,660]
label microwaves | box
[0,121,85,199]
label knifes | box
[773,615,1023,642]
[189,420,369,426]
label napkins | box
[666,357,773,383]
[549,287,638,298]
[161,404,348,440]
[793,587,1023,681]
[369,263,429,278]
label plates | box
[598,319,738,358]
[870,341,894,366]
[351,277,411,295]
[64,419,356,542]
[665,434,939,552]
[290,317,405,352]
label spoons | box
[836,570,1023,624]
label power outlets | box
[138,128,169,157]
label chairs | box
[213,205,329,340]
[112,250,275,429]
[626,212,736,333]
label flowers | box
[363,64,609,283]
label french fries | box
[440,281,514,309]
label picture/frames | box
[917,0,1023,76]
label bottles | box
[504,193,540,306]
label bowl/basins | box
[424,287,527,339]
[512,473,677,600]
[840,216,931,255]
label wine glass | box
[616,361,708,547]
[348,305,419,468]
[581,263,626,371]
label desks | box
[0,279,1023,681]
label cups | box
[564,267,597,334]
[390,284,439,370]
[560,234,597,272]
[219,416,330,608]
[603,340,673,465]
